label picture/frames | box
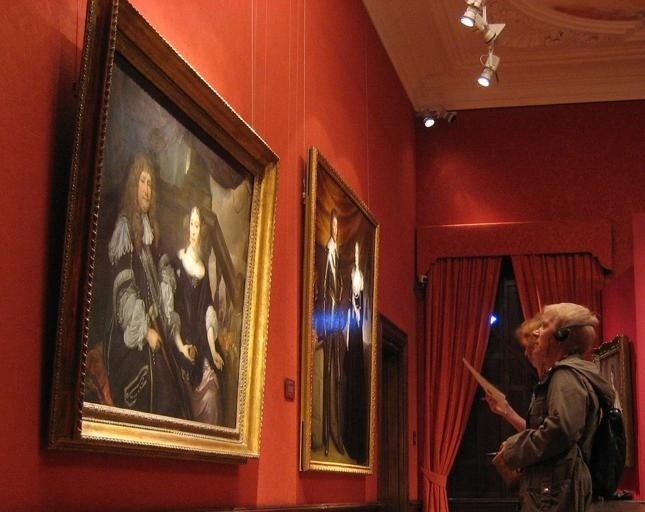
[591,334,635,467]
[298,145,381,476]
[41,0,280,466]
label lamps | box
[439,107,457,124]
[420,108,435,128]
[477,55,500,87]
[460,0,486,27]
[472,12,506,44]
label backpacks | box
[591,403,628,495]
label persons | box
[105,152,180,418]
[318,208,345,456]
[609,363,621,412]
[484,311,544,433]
[342,239,369,465]
[491,302,616,511]
[158,202,225,425]
